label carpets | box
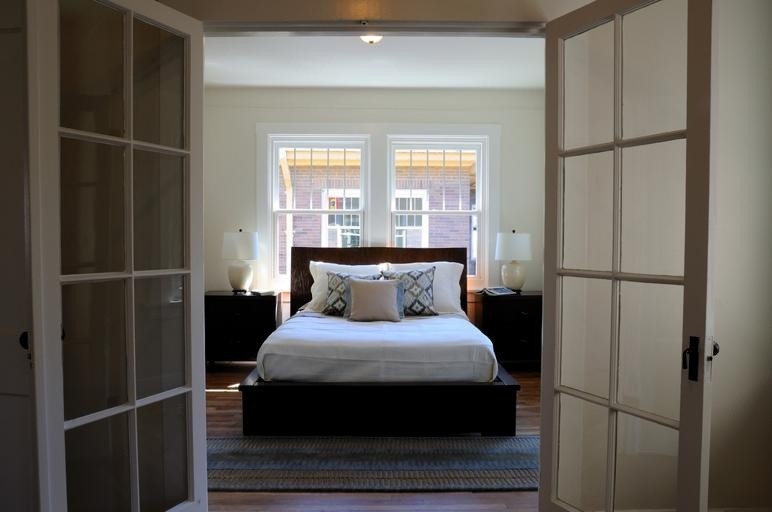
[206,435,541,494]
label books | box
[472,286,517,297]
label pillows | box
[343,277,405,319]
[321,272,382,316]
[380,266,439,316]
[300,259,382,314]
[378,261,469,320]
[349,276,402,322]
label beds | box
[238,245,523,436]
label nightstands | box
[205,289,281,370]
[466,289,543,373]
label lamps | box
[221,227,260,294]
[493,229,535,294]
